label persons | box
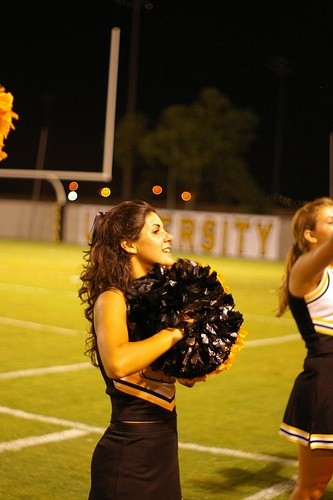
[277,197,333,500]
[81,199,193,500]
